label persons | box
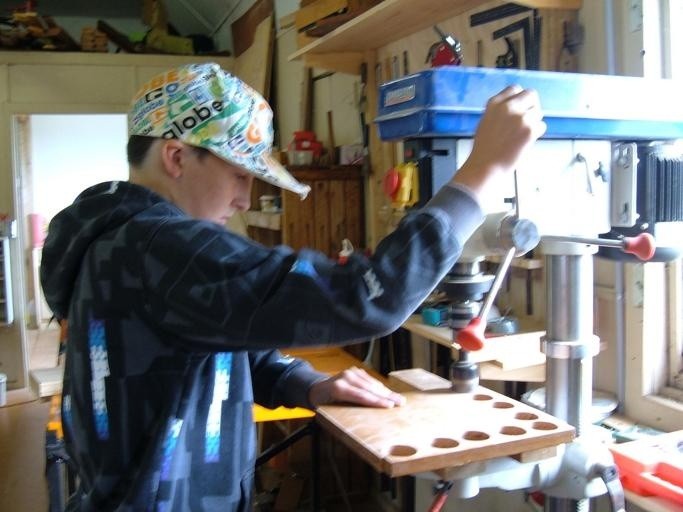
[39,62,547,511]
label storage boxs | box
[294,1,366,37]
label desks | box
[46,343,390,511]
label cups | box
[259,195,279,212]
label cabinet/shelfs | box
[245,167,362,254]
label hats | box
[126,62,312,202]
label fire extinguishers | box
[424,25,466,67]
